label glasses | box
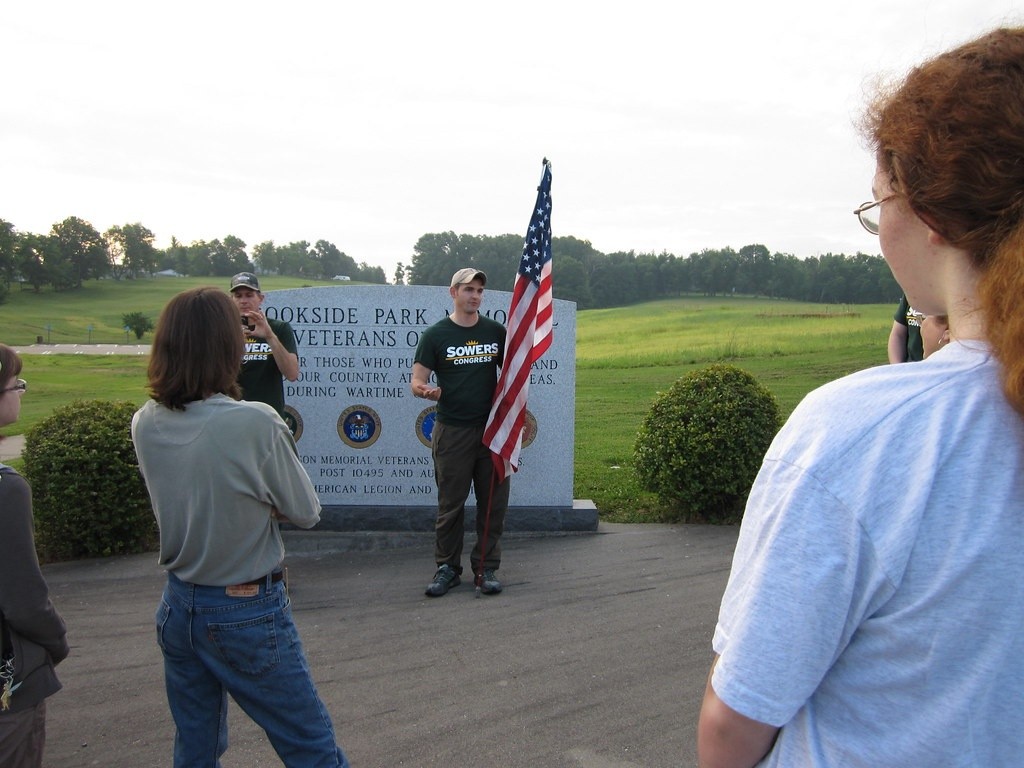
[854,193,906,234]
[2,378,27,396]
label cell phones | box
[241,316,248,325]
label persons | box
[0,344,69,768]
[410,267,511,596]
[697,23,1024,768]
[224,272,300,424]
[131,288,351,768]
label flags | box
[483,161,554,483]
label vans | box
[333,275,350,280]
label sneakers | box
[426,564,462,596]
[474,569,503,595]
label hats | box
[229,272,261,292]
[450,267,488,288]
